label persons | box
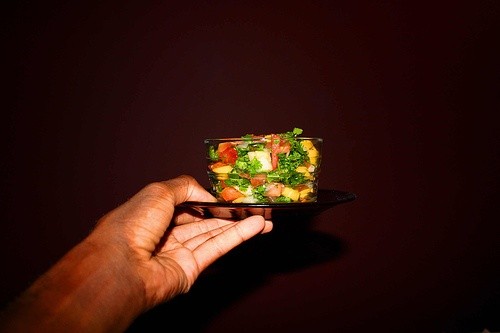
[0,176,273,331]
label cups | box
[202,136,324,202]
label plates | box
[178,189,356,220]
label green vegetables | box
[208,127,310,203]
